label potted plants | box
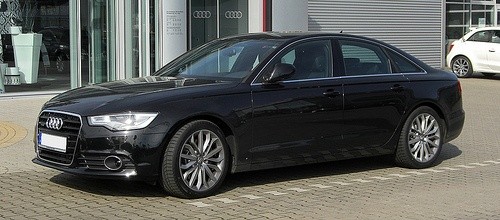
[11,0,42,84]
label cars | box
[446,27,500,78]
[37,29,88,63]
[32,31,466,200]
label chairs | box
[250,47,385,78]
